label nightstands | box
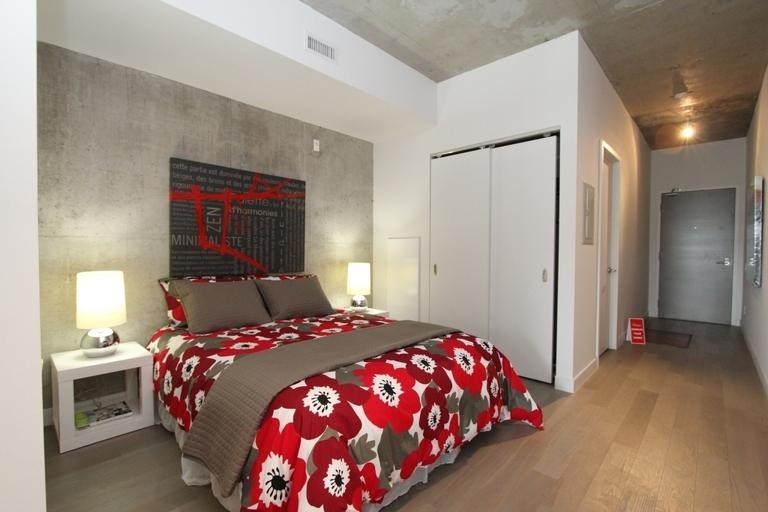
[50,341,156,454]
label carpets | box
[645,328,693,348]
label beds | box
[146,308,545,512]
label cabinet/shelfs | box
[427,125,562,387]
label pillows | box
[157,271,336,335]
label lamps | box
[345,262,372,309]
[75,270,128,359]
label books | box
[83,399,134,428]
